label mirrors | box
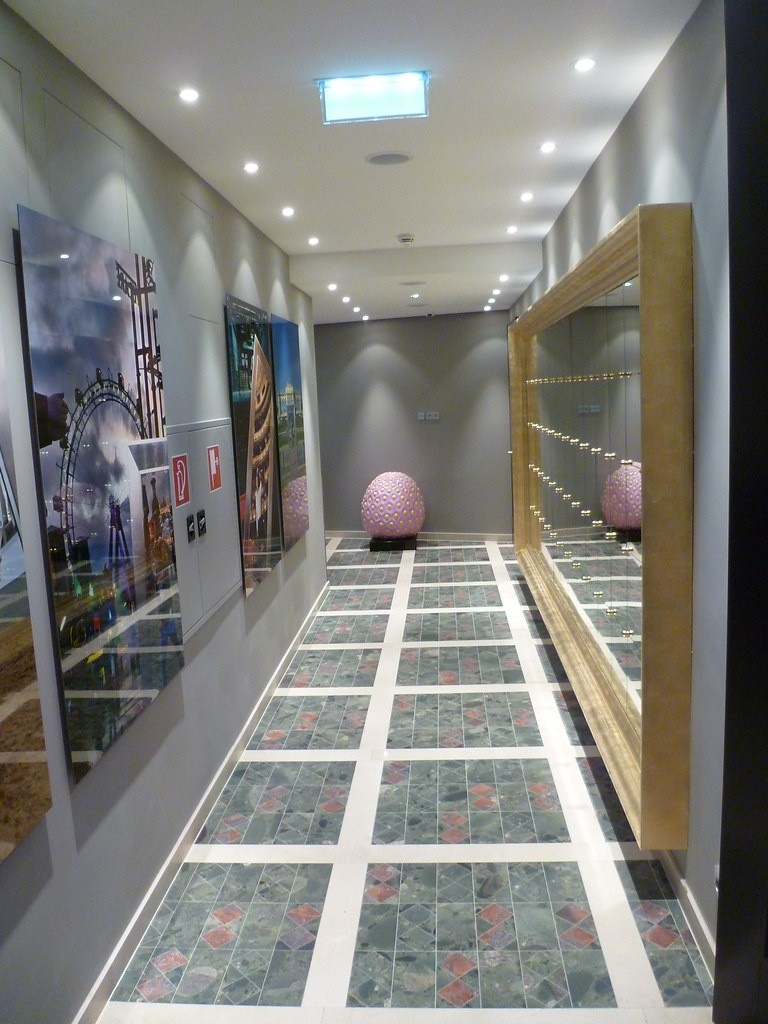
[507,203,691,851]
